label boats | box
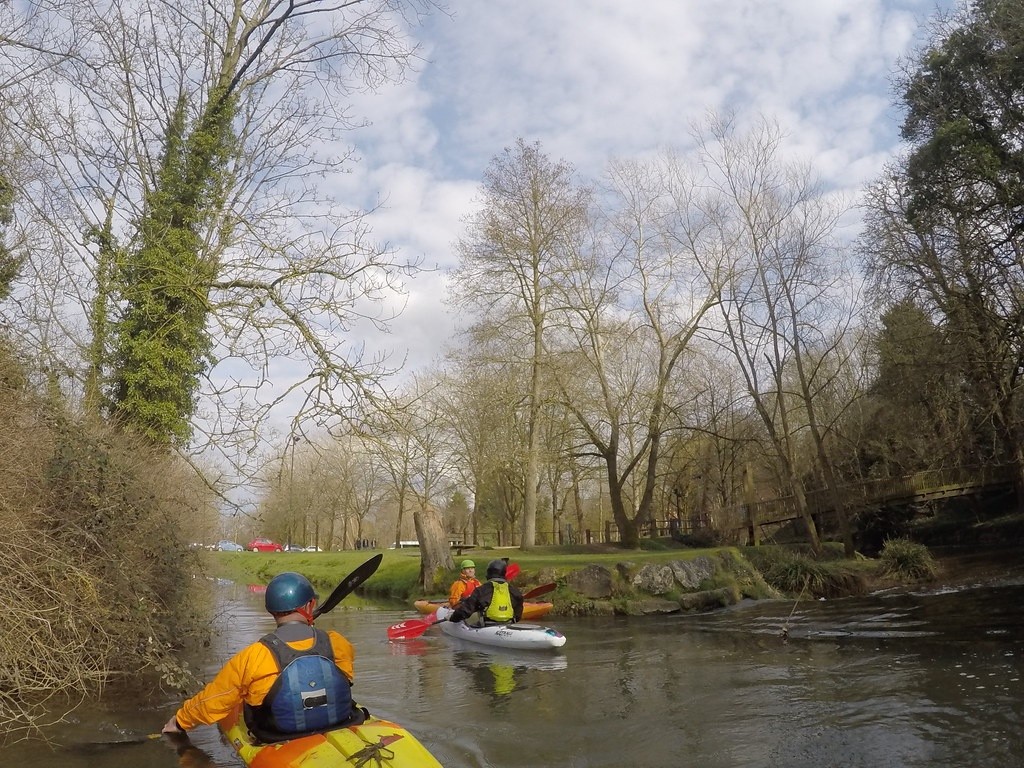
[414,600,554,620]
[216,702,442,768]
[436,608,567,648]
[437,633,569,671]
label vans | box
[386,541,420,548]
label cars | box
[205,543,218,551]
[247,537,284,553]
[283,545,308,553]
[302,545,323,553]
[215,540,244,553]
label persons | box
[451,558,523,629]
[356,537,377,550]
[166,571,358,738]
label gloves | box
[450,610,467,623]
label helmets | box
[264,572,320,626]
[486,560,507,578]
[461,560,476,570]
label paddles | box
[425,563,520,624]
[71,554,385,748]
[387,582,557,640]
